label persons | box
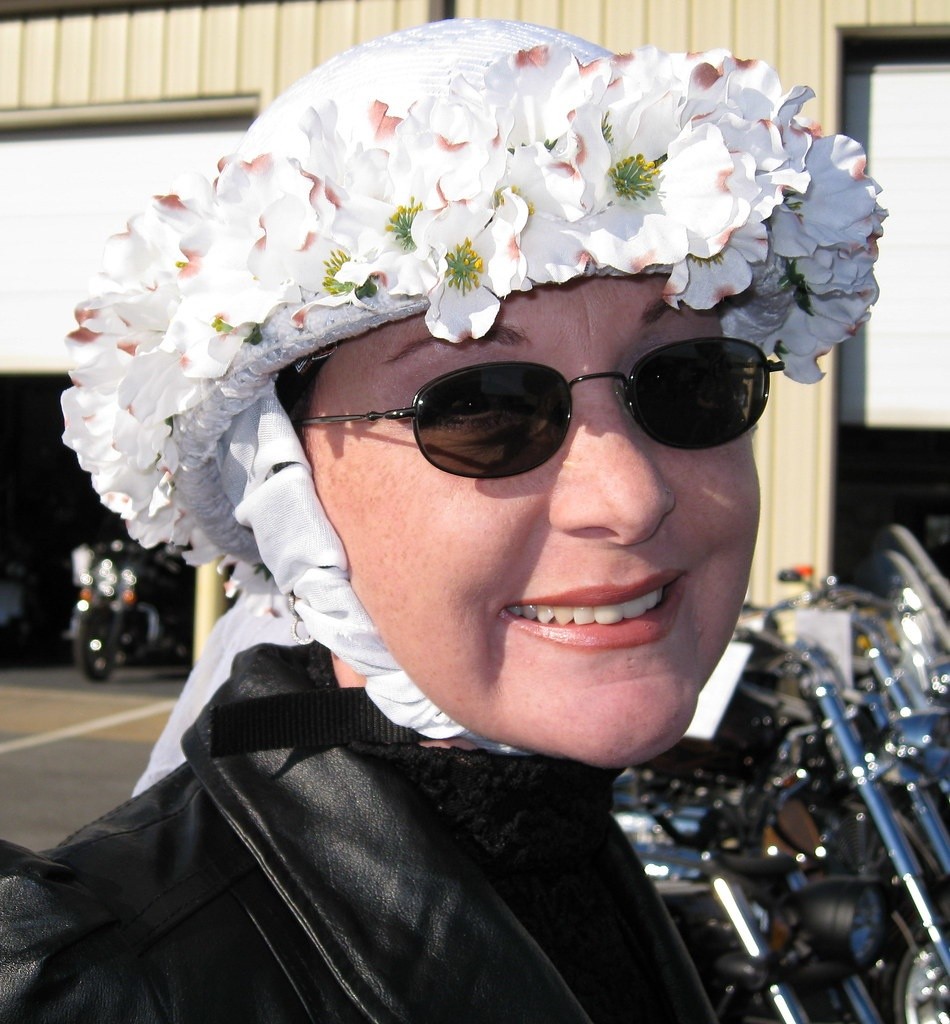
[0,20,786,1024]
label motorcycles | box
[65,525,194,686]
[602,518,950,1023]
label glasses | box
[294,336,786,480]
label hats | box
[61,21,891,773]
